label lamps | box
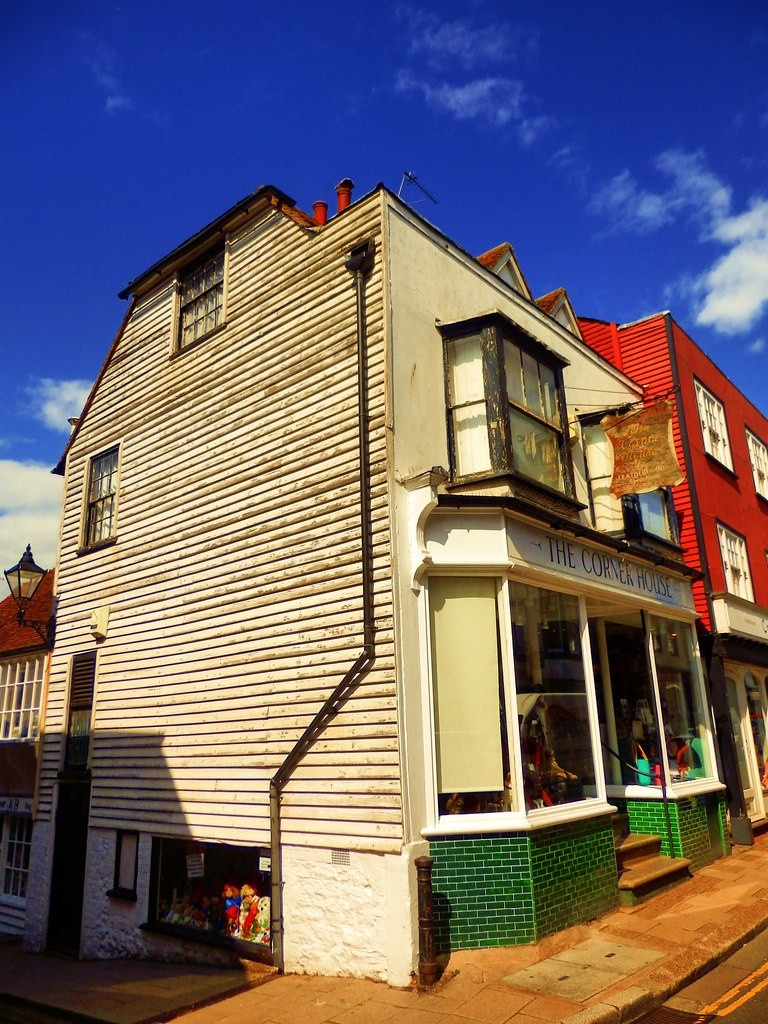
[4,542,58,653]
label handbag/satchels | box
[521,714,547,755]
[633,741,651,785]
[543,771,586,804]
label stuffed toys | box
[162,883,271,943]
